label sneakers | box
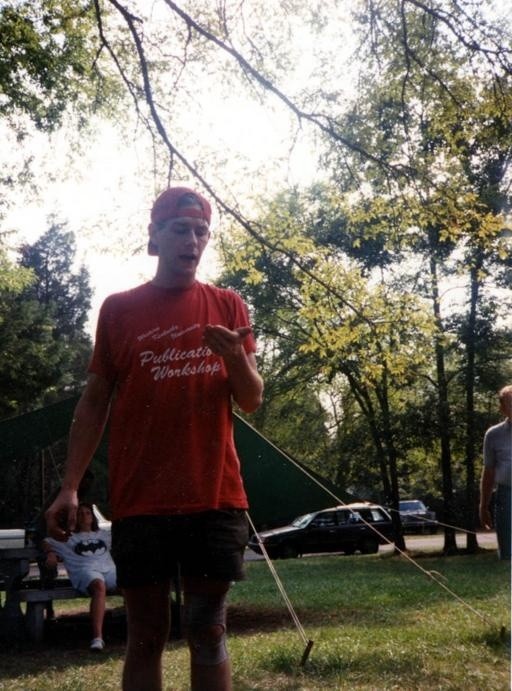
[90,640,105,651]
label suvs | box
[248,504,394,560]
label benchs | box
[0,548,121,648]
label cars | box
[384,500,438,535]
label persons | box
[26,504,127,653]
[477,385,510,568]
[42,185,264,690]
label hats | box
[147,187,211,255]
